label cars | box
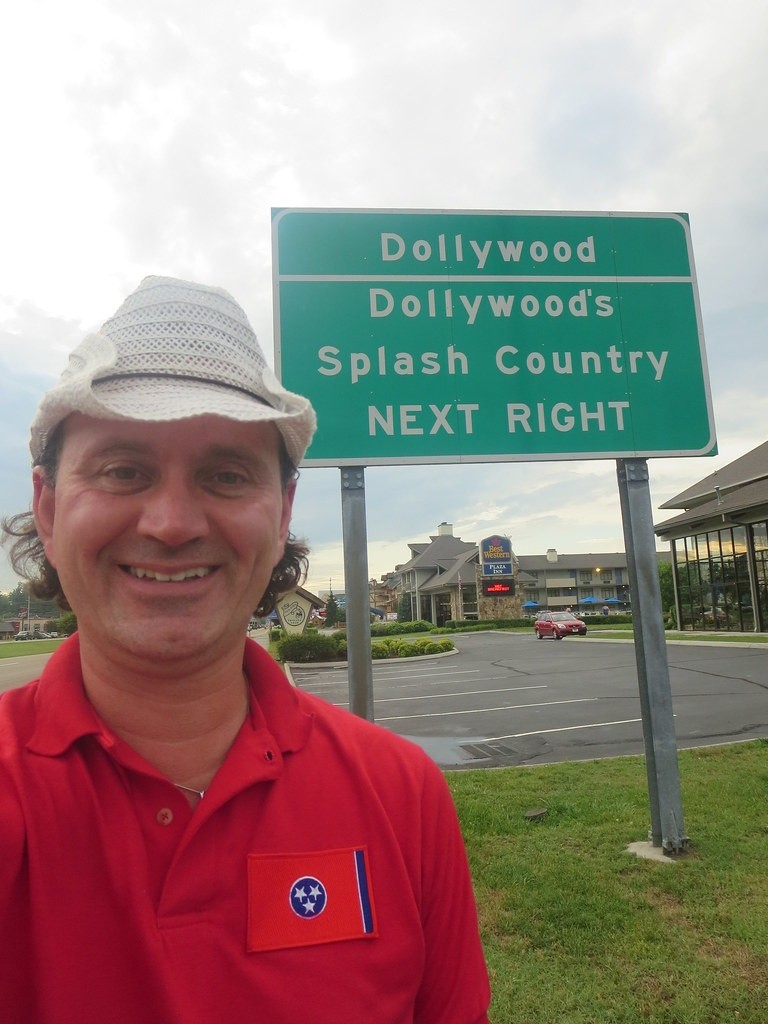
[247,620,282,631]
[735,602,752,613]
[697,606,734,625]
[533,611,588,639]
[12,631,69,641]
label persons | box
[0,275,492,1024]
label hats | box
[28,274,318,470]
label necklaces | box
[172,781,208,801]
